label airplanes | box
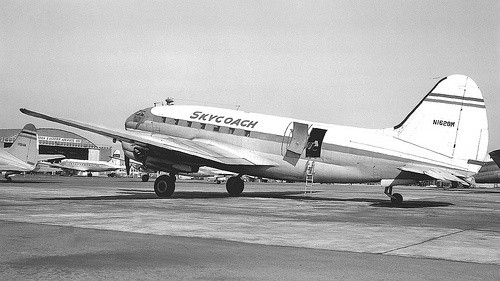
[49,149,126,178]
[0,123,66,182]
[108,155,258,184]
[18,73,494,205]
